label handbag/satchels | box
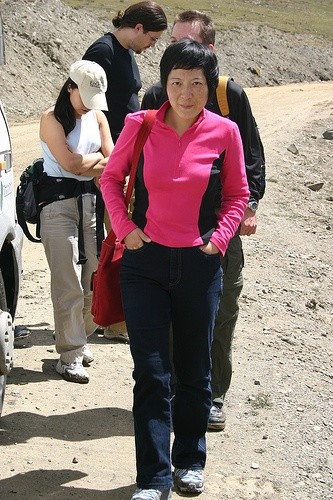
[89,240,126,328]
[14,157,103,243]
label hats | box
[70,60,109,112]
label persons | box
[99,40,252,500]
[126,8,265,433]
[79,0,167,290]
[35,60,114,384]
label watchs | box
[247,201,259,211]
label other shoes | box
[207,402,226,430]
[55,359,89,383]
[173,467,204,493]
[52,331,94,363]
[131,484,173,500]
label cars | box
[0,98,25,423]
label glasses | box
[147,32,158,45]
